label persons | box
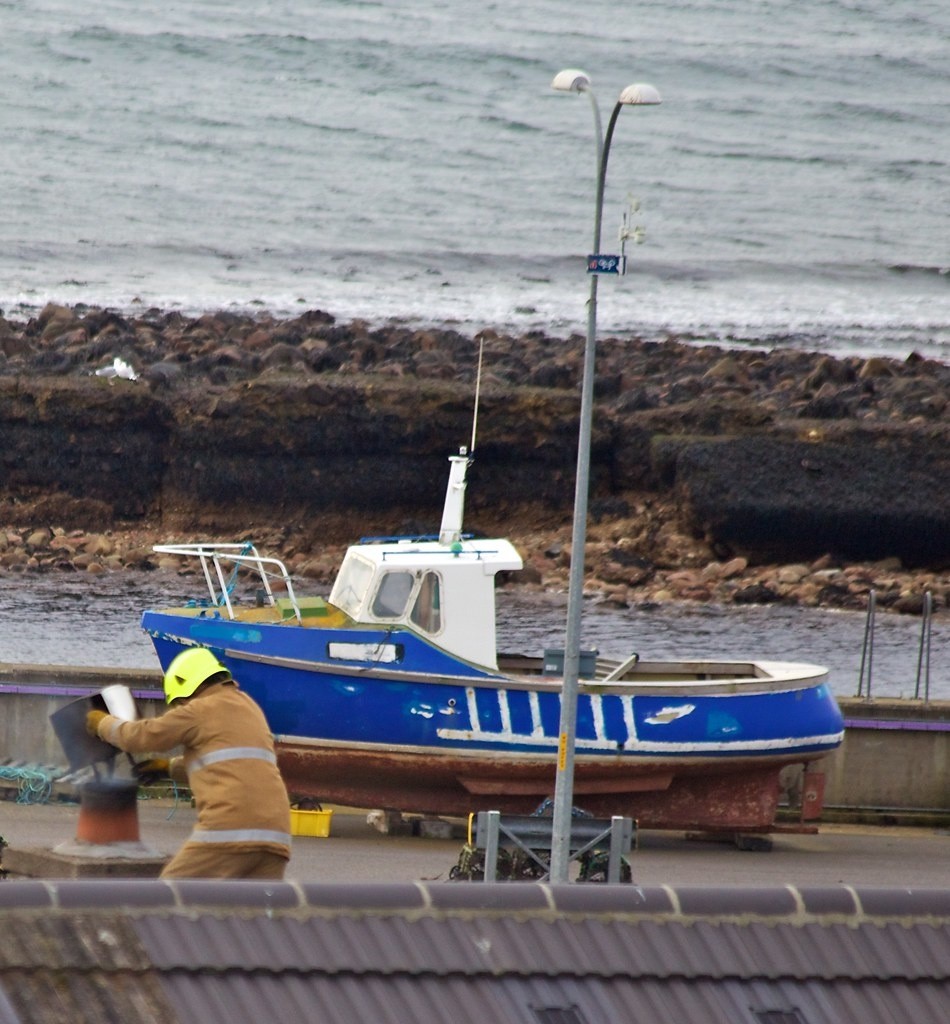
[86,647,291,881]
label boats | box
[138,338,848,833]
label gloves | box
[132,757,169,786]
[86,710,108,735]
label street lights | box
[548,69,666,885]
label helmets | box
[164,646,232,704]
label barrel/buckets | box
[542,650,597,681]
[48,685,148,771]
